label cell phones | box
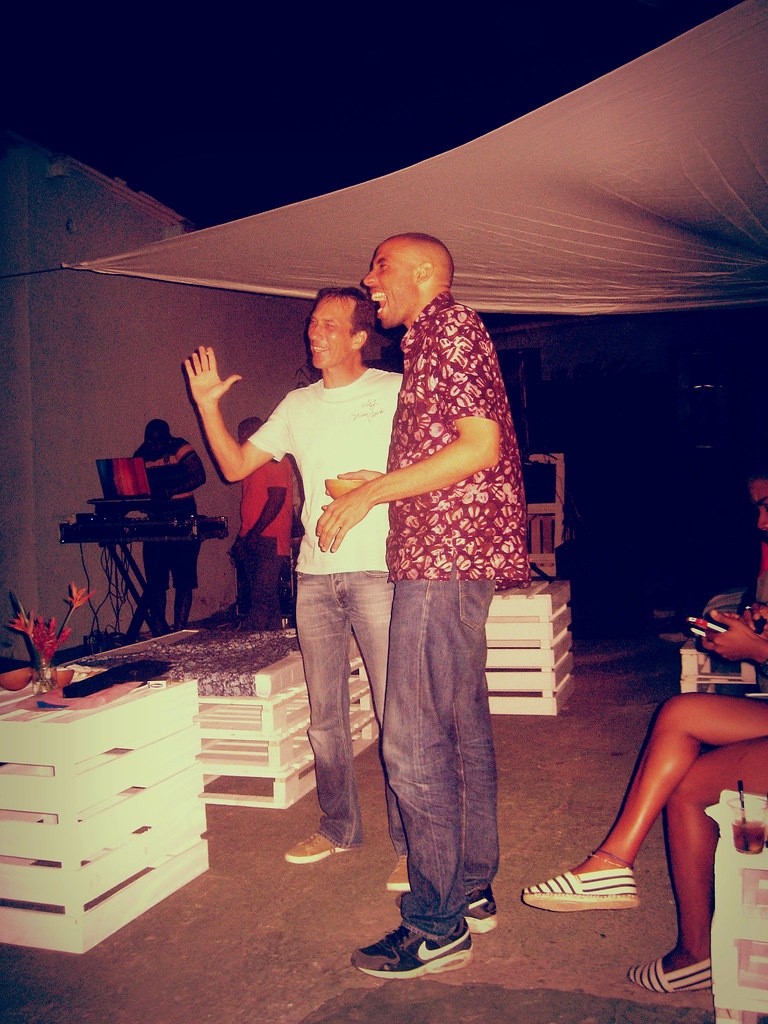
[687,617,727,633]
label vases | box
[31,657,57,695]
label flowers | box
[6,581,95,657]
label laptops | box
[95,457,151,498]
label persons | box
[227,416,294,632]
[182,288,410,893]
[129,417,207,634]
[523,445,768,991]
[312,232,528,981]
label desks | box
[486,581,574,715]
[1,663,212,955]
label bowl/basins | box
[1,667,32,691]
[325,479,366,500]
[46,670,74,688]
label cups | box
[728,797,766,854]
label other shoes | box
[626,949,712,994]
[522,866,639,912]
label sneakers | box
[350,922,475,979]
[462,881,497,934]
[385,854,411,893]
[283,830,351,864]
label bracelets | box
[762,658,768,666]
